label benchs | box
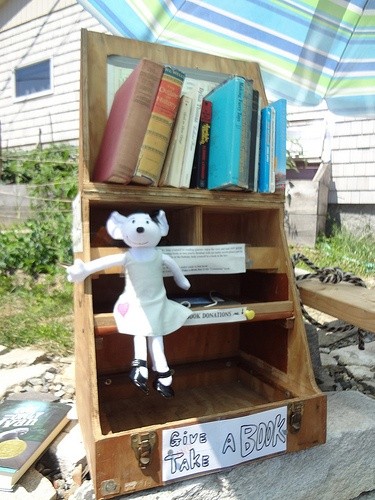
[293,267,375,333]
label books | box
[0,400,73,491]
[92,58,287,194]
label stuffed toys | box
[67,209,194,399]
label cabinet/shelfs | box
[72,28,328,500]
